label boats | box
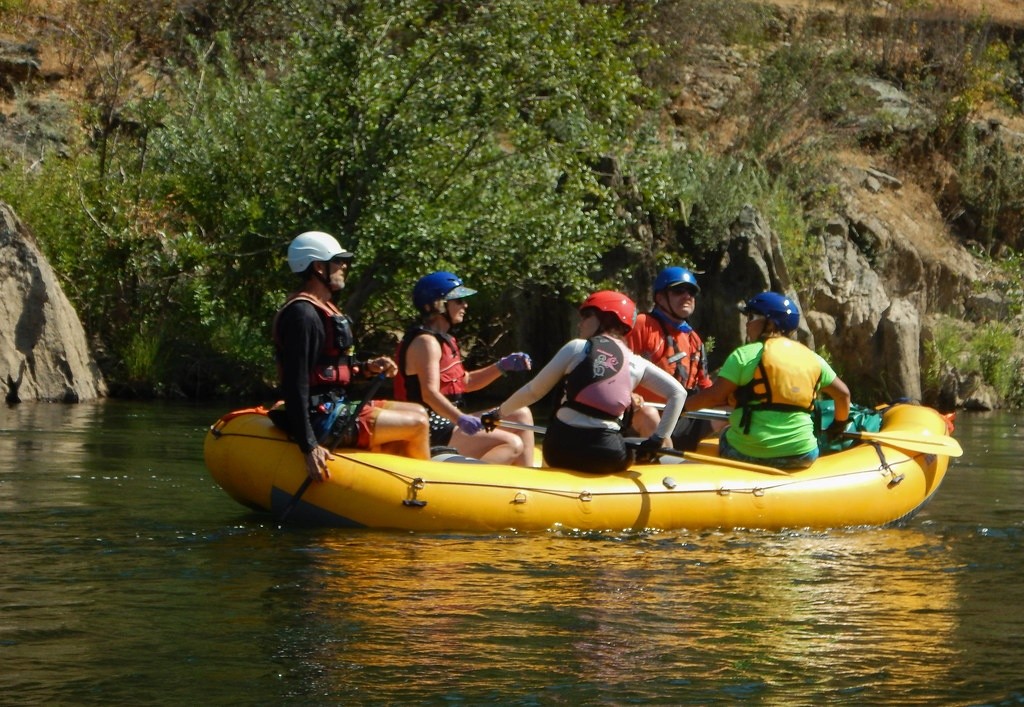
[203,405,954,533]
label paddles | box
[484,417,790,478]
[641,401,964,458]
[276,358,388,529]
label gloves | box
[827,419,848,443]
[458,414,484,436]
[635,434,663,460]
[481,410,501,433]
[500,352,532,372]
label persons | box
[274,230,431,483]
[481,289,688,475]
[396,270,538,467]
[678,290,851,469]
[624,266,727,452]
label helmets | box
[578,291,636,336]
[653,267,697,290]
[748,292,799,329]
[287,231,346,272]
[413,272,463,312]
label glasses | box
[583,310,595,318]
[747,313,766,322]
[667,285,697,297]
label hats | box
[442,285,478,300]
[670,281,701,295]
[334,251,355,258]
[737,306,761,314]
